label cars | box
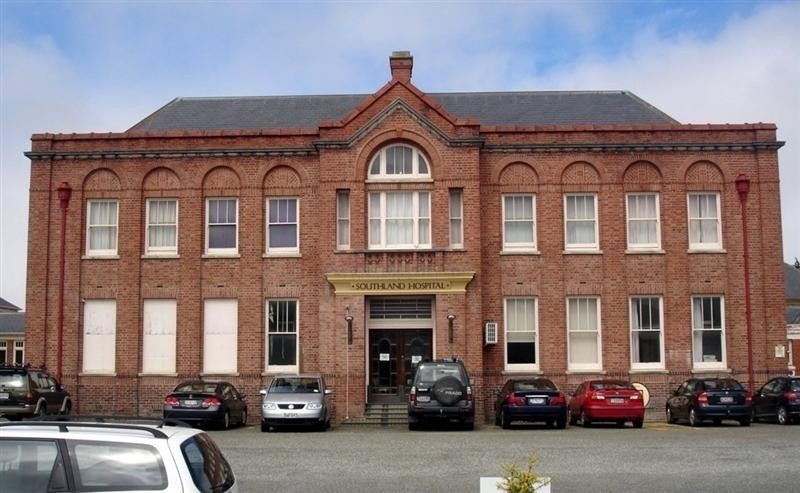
[163,380,248,430]
[567,378,645,428]
[752,376,800,424]
[665,377,753,427]
[493,377,568,430]
[260,373,334,431]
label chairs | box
[277,383,292,392]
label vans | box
[0,413,240,492]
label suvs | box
[406,358,476,429]
[0,362,73,420]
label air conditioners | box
[485,323,498,344]
